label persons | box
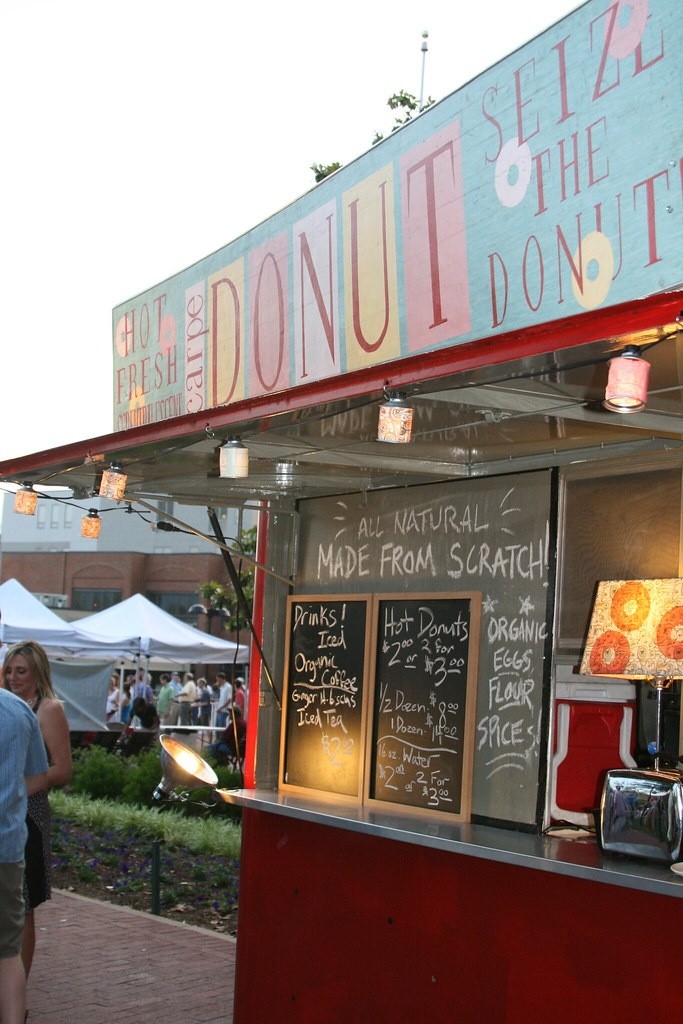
[0,640,72,980]
[214,672,233,729]
[215,706,247,761]
[105,672,213,726]
[118,697,159,743]
[0,686,49,1024]
[233,677,245,718]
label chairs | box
[227,732,246,774]
[106,721,126,755]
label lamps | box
[217,436,249,479]
[374,390,414,444]
[98,462,128,501]
[152,732,219,807]
[13,481,38,517]
[602,344,651,417]
[81,507,102,539]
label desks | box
[128,725,227,753]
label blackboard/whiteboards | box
[278,465,566,837]
[363,590,483,824]
[276,593,374,813]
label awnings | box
[1,292,682,586]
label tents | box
[69,593,250,732]
[0,578,140,731]
